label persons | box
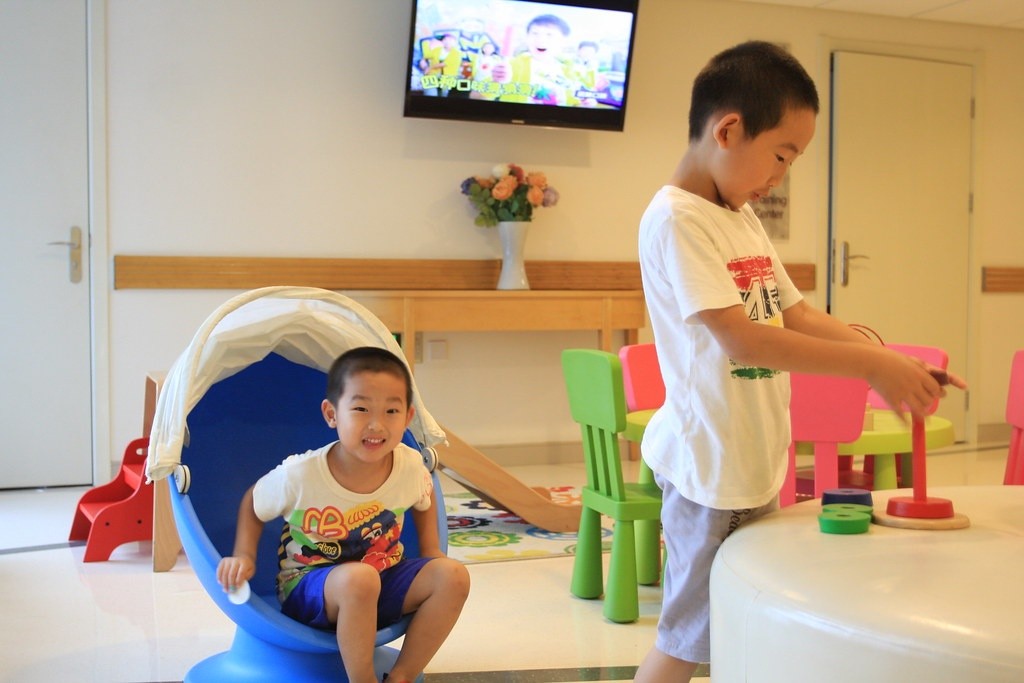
[420,14,598,110]
[632,40,969,683]
[216,346,471,683]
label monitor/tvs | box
[403,0,640,133]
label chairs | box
[559,345,662,623]
[618,344,666,414]
[1002,348,1024,486]
[836,343,950,476]
[776,371,871,508]
[143,284,449,683]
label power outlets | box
[429,339,446,360]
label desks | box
[621,405,954,585]
[708,481,1024,683]
[332,286,645,462]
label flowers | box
[459,160,559,228]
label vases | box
[495,221,531,290]
